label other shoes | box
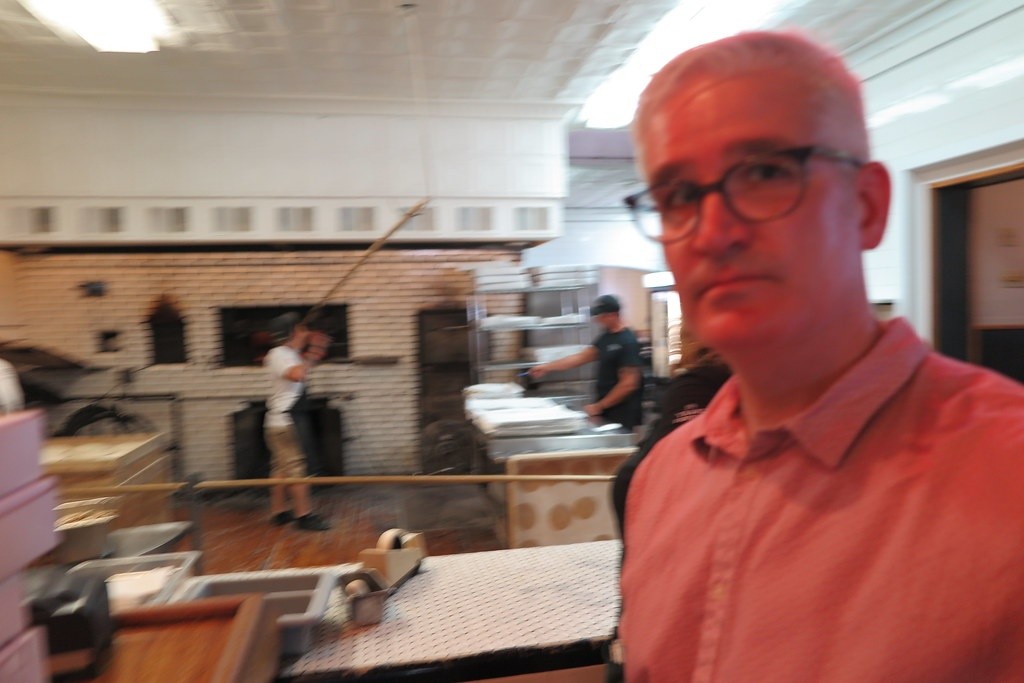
[272,510,293,526]
[296,515,328,531]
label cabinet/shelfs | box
[466,282,599,470]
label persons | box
[615,30,1024,683]
[607,313,738,683]
[265,313,335,528]
[527,294,644,430]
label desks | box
[39,430,174,556]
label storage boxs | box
[0,408,61,683]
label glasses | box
[621,145,874,243]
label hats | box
[584,294,621,315]
